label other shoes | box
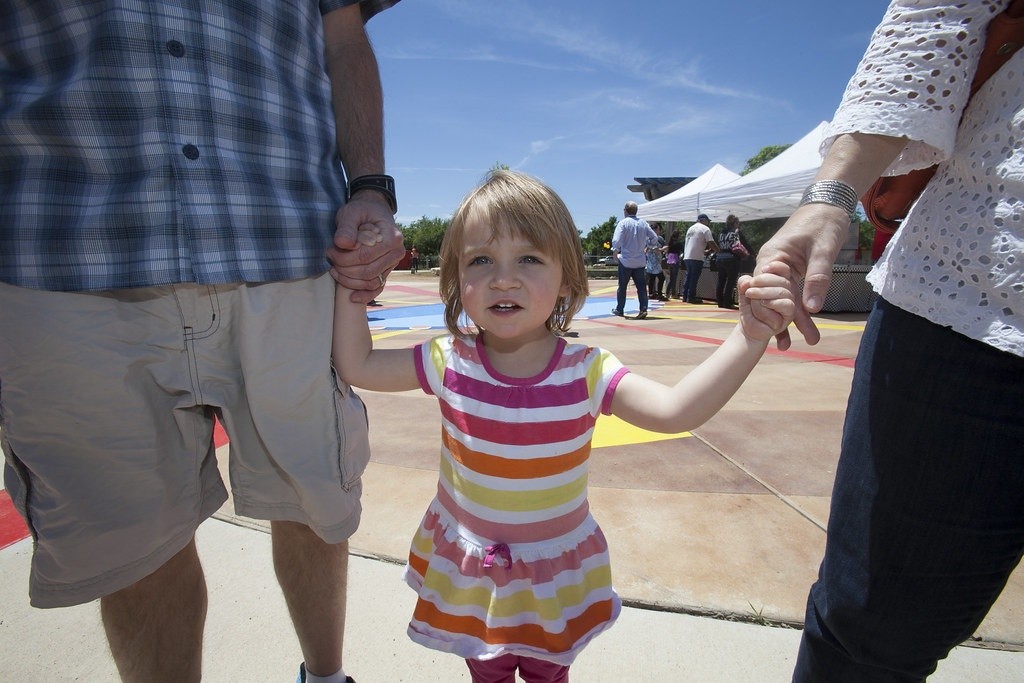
[657,295,668,301]
[612,308,623,316]
[296,662,357,683]
[636,310,647,319]
[717,303,739,309]
[648,293,657,298]
[683,297,702,303]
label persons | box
[744,0,1024,683]
[611,201,752,319]
[0,0,404,683]
[332,167,796,683]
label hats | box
[698,214,712,221]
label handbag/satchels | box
[858,167,930,235]
[710,253,719,271]
[740,252,756,273]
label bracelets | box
[347,175,398,212]
[799,180,859,216]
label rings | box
[378,274,384,287]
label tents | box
[635,120,829,223]
